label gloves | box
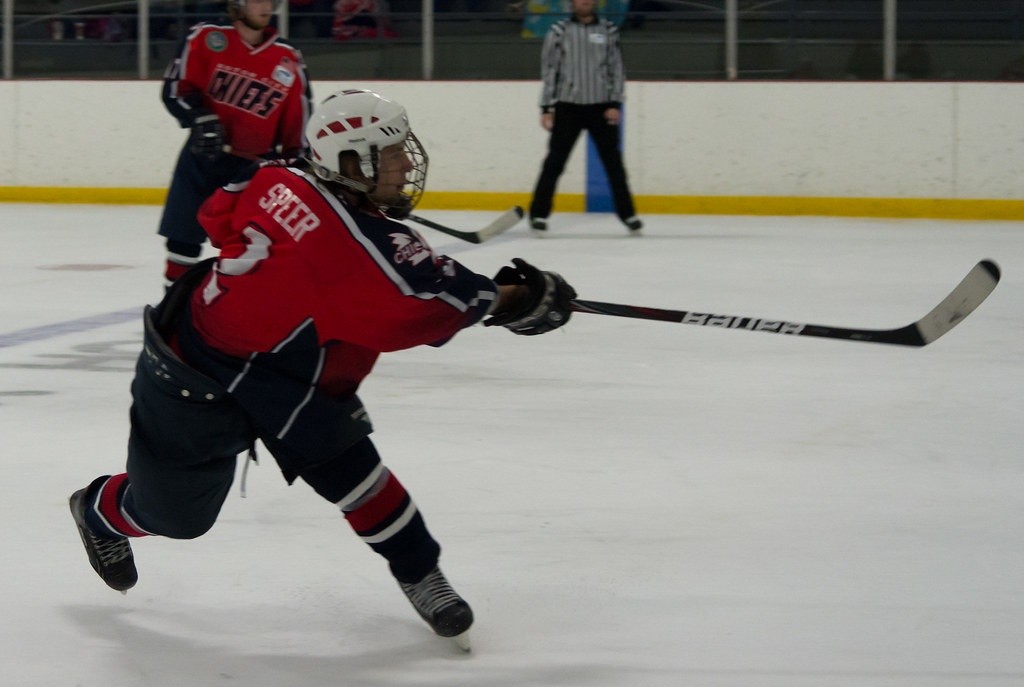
[483,258,576,336]
[189,111,229,163]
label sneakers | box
[531,219,547,235]
[70,486,138,590]
[398,563,474,638]
[626,216,644,237]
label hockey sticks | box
[221,140,525,244]
[566,259,1001,347]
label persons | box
[156,1,315,292]
[68,86,579,638]
[527,0,645,235]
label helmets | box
[228,0,282,21]
[305,89,430,221]
